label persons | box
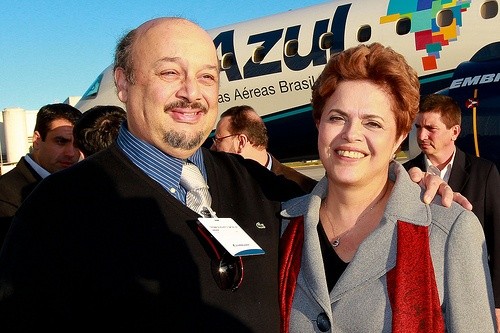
[73,106,127,158]
[402,95,500,333]
[278,42,498,332]
[0,17,472,333]
[209,105,318,195]
[1,103,82,248]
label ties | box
[178,163,212,219]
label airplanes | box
[72,1,500,183]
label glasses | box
[212,134,240,146]
[197,224,243,293]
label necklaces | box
[325,190,388,248]
